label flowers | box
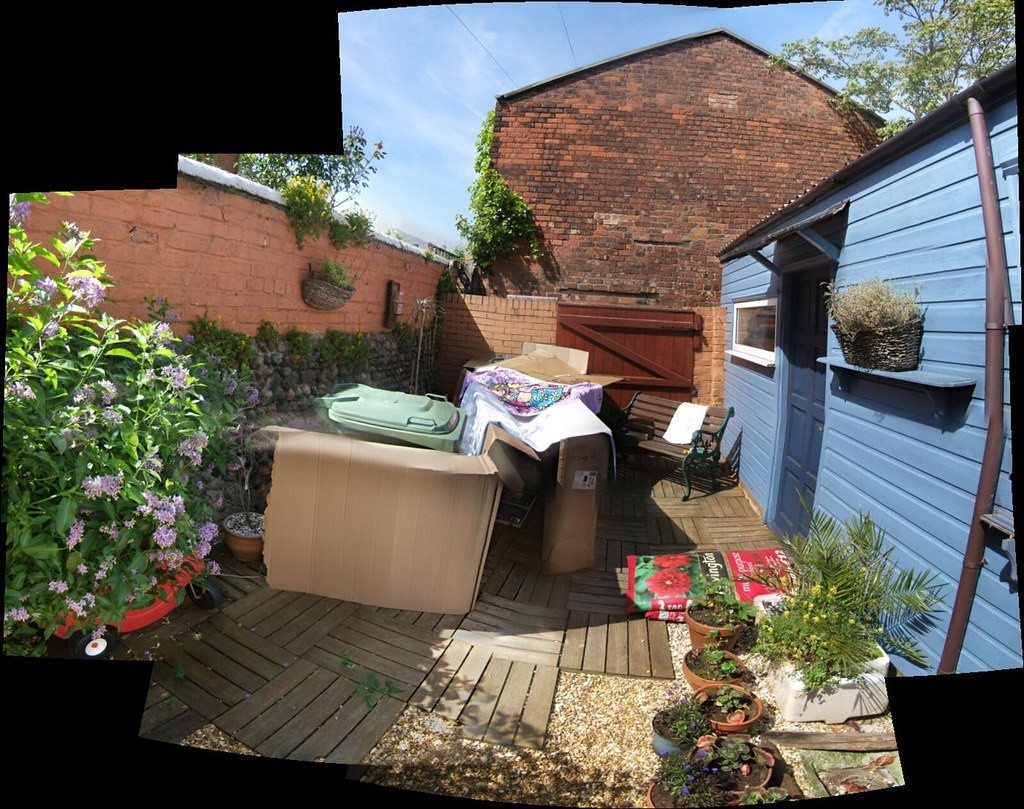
[650,746,733,808]
[3,190,261,656]
[654,681,709,743]
[731,482,951,697]
[695,733,775,777]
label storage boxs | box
[535,434,608,576]
[259,415,542,616]
[480,422,541,529]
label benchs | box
[601,391,735,502]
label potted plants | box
[691,684,762,736]
[683,629,746,692]
[686,576,758,652]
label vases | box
[647,778,737,809]
[686,736,772,792]
[651,709,695,757]
[754,592,891,726]
[223,512,264,564]
